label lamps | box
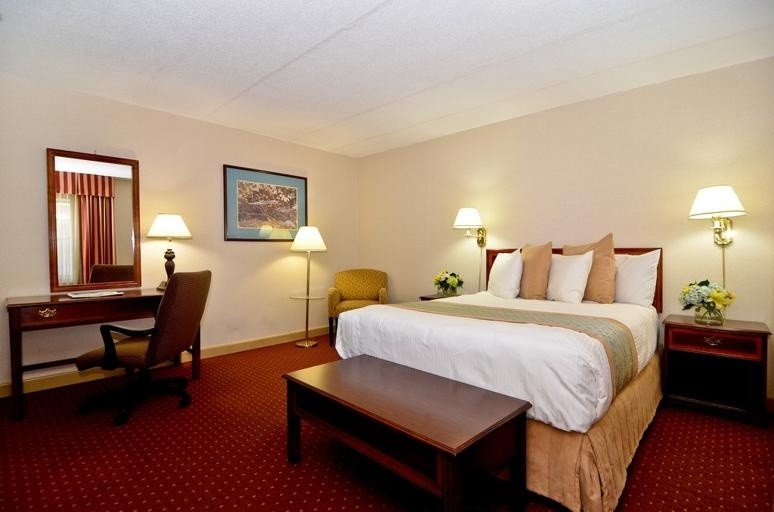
[452,205,487,247]
[289,222,327,349]
[687,183,747,248]
[146,211,191,292]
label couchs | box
[327,268,390,349]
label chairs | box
[73,268,214,428]
[85,260,135,285]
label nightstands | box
[418,293,461,300]
[661,312,773,427]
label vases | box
[693,305,729,325]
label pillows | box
[560,231,619,306]
[518,241,553,302]
[546,249,595,306]
[485,246,524,300]
[611,246,664,308]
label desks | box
[279,350,531,510]
[1,285,203,420]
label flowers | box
[678,279,738,314]
[432,262,464,295]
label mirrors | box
[44,145,142,292]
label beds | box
[334,240,671,511]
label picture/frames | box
[219,163,308,244]
